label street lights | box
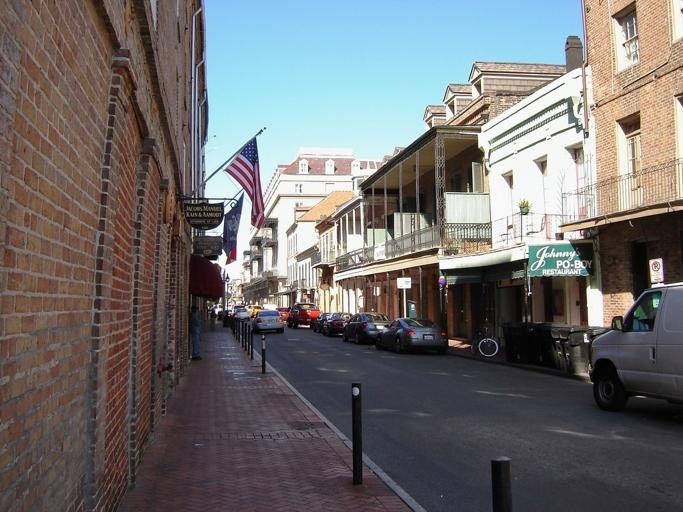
[224,272,230,316]
[289,281,293,307]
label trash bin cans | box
[501,321,609,373]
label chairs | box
[498,224,518,249]
[526,213,547,240]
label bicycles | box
[469,324,499,358]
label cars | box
[320,312,354,336]
[313,313,332,333]
[209,302,277,321]
[251,309,285,335]
[340,312,392,346]
[375,317,449,354]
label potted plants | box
[517,199,533,215]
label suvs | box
[285,302,321,329]
[276,307,289,321]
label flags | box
[223,136,264,229]
[223,192,244,265]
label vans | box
[585,280,682,416]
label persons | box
[189,305,203,360]
